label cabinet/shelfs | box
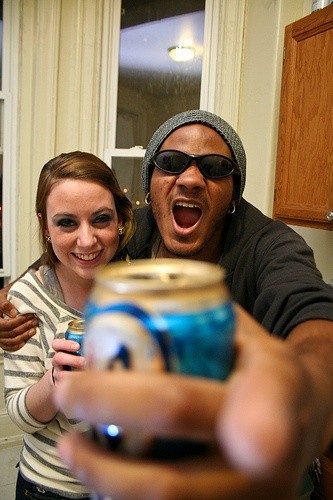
[272,2,333,232]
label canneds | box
[65,319,87,371]
[83,258,236,461]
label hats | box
[141,109,246,206]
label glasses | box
[148,149,239,180]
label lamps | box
[168,46,196,62]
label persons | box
[2,151,137,500]
[0,110,333,500]
